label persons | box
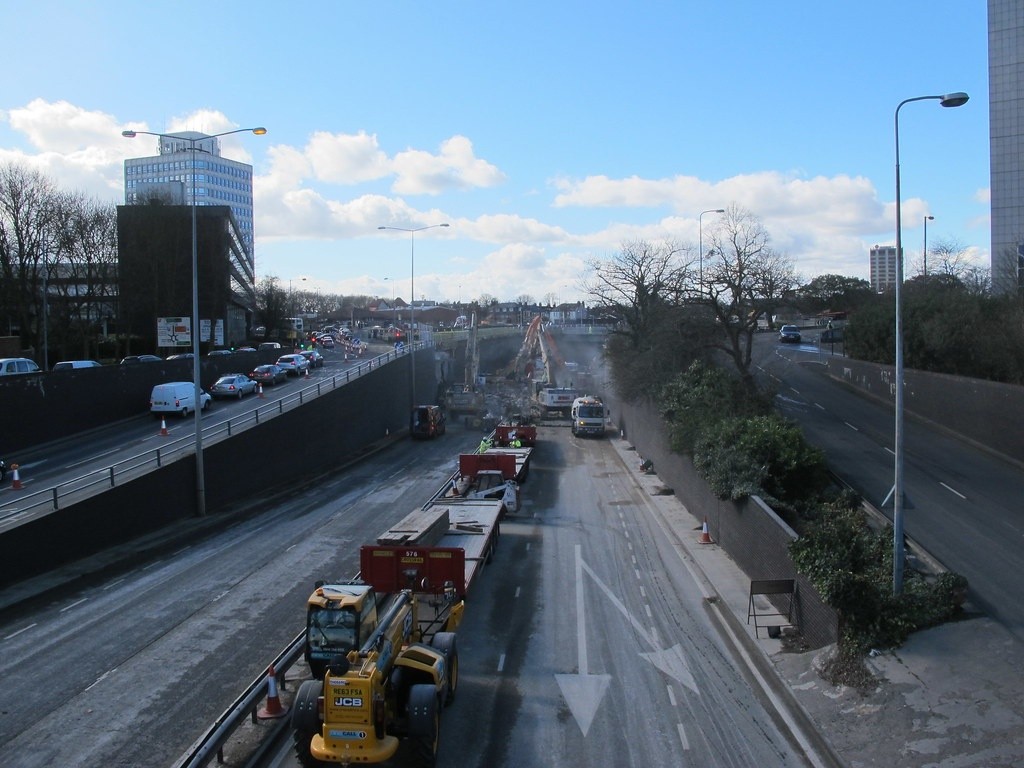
[480,437,491,454]
[509,436,521,448]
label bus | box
[284,318,302,332]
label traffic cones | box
[9,464,27,490]
[607,410,613,425]
[618,429,626,441]
[637,456,647,473]
[698,515,716,544]
[304,369,311,379]
[257,386,266,398]
[344,346,366,362]
[449,476,462,497]
[157,415,170,435]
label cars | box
[780,324,802,342]
[0,357,102,377]
[310,324,419,348]
[121,342,323,419]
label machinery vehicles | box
[570,395,606,437]
[290,422,539,768]
[444,312,580,427]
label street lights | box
[289,279,307,346]
[379,223,449,405]
[923,216,935,285]
[700,209,724,303]
[893,91,971,592]
[42,218,75,371]
[120,129,269,518]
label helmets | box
[511,436,516,439]
[483,437,487,441]
